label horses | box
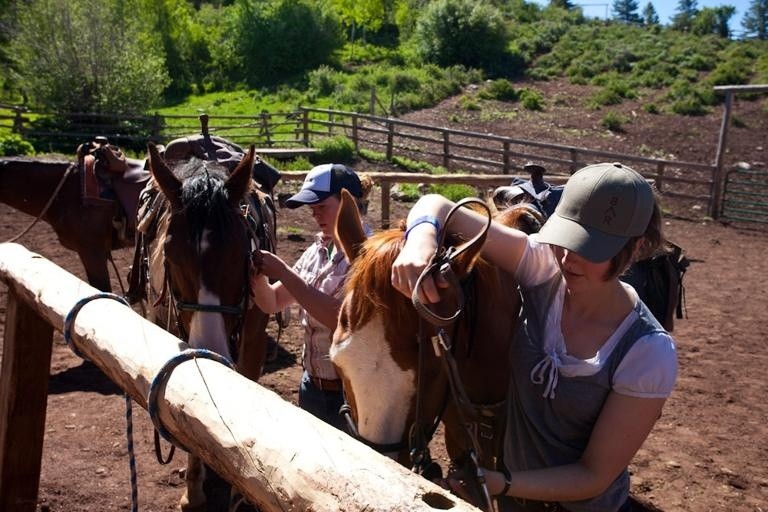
[147,142,276,512]
[0,156,151,386]
[321,188,679,473]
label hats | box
[534,162,655,264]
[285,162,363,210]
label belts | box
[307,375,340,392]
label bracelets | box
[492,466,512,497]
[404,215,441,243]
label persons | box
[250,161,375,433]
[390,162,678,512]
